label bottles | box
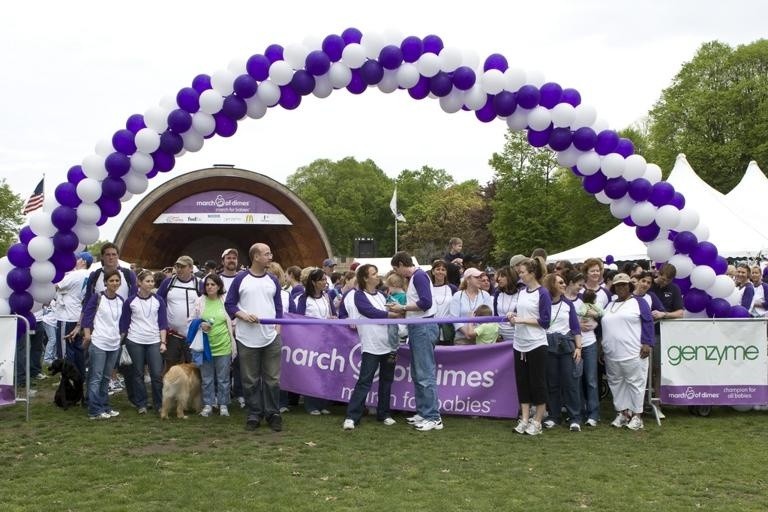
[203,315,216,335]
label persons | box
[18,243,768,436]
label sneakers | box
[406,414,443,431]
[245,420,259,430]
[309,408,330,416]
[36,374,47,380]
[343,418,355,429]
[652,407,666,420]
[129,402,152,414]
[280,407,289,412]
[383,418,396,425]
[267,413,281,432]
[199,396,245,418]
[514,402,600,436]
[89,378,125,420]
[611,409,643,430]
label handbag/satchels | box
[54,363,83,409]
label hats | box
[175,256,193,267]
[510,255,529,266]
[322,259,338,267]
[202,260,217,268]
[76,252,94,264]
[612,272,630,284]
[464,255,495,278]
[222,248,239,258]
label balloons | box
[1,28,753,332]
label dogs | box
[160,362,205,420]
[48,359,88,411]
